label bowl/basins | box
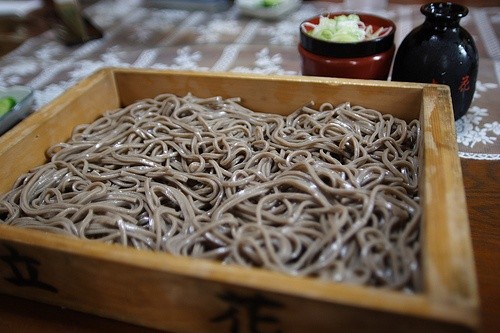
[300,11,395,58]
[298,44,396,81]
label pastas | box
[1,92,426,295]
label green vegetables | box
[301,12,392,43]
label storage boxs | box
[0,65,480,333]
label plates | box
[0,86,33,133]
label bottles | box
[392,2,479,122]
